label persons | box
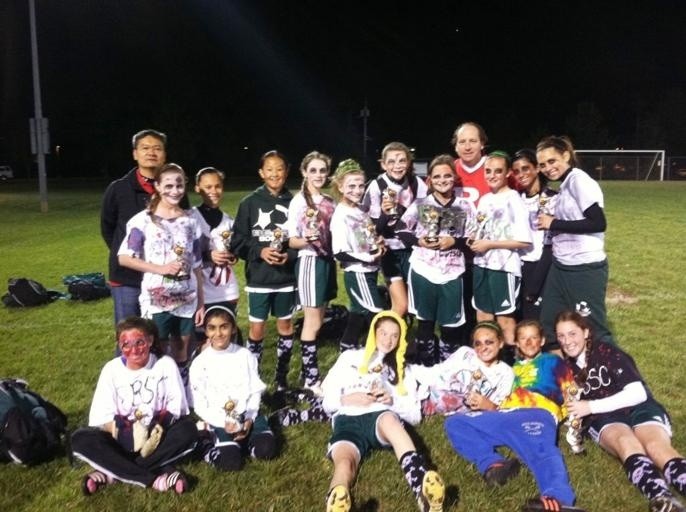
[99,129,337,407]
[329,122,617,367]
[69,306,281,494]
[317,309,686,511]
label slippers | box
[484,458,519,488]
[522,495,583,512]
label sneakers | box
[299,377,322,397]
[189,430,213,460]
[152,469,190,494]
[326,485,352,512]
[273,378,287,391]
[81,470,107,496]
[416,470,446,512]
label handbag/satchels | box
[296,304,349,341]
[0,377,69,468]
[1,279,59,309]
[60,272,111,301]
